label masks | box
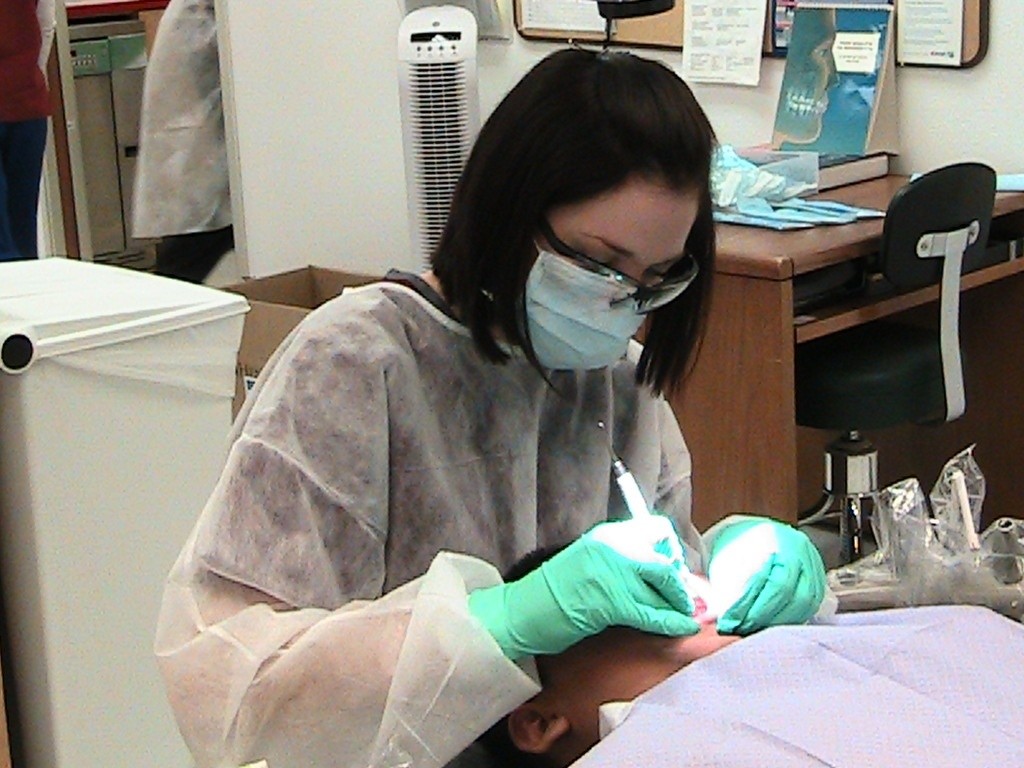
[516,239,648,372]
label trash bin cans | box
[0,253,255,768]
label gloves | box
[467,512,701,662]
[708,514,826,637]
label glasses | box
[537,216,698,315]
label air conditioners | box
[397,5,480,273]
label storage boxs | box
[737,149,888,196]
[223,265,384,424]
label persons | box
[473,547,743,768]
[131,0,237,285]
[0,0,57,262]
[154,50,830,768]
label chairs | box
[795,162,995,564]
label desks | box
[634,175,1024,535]
